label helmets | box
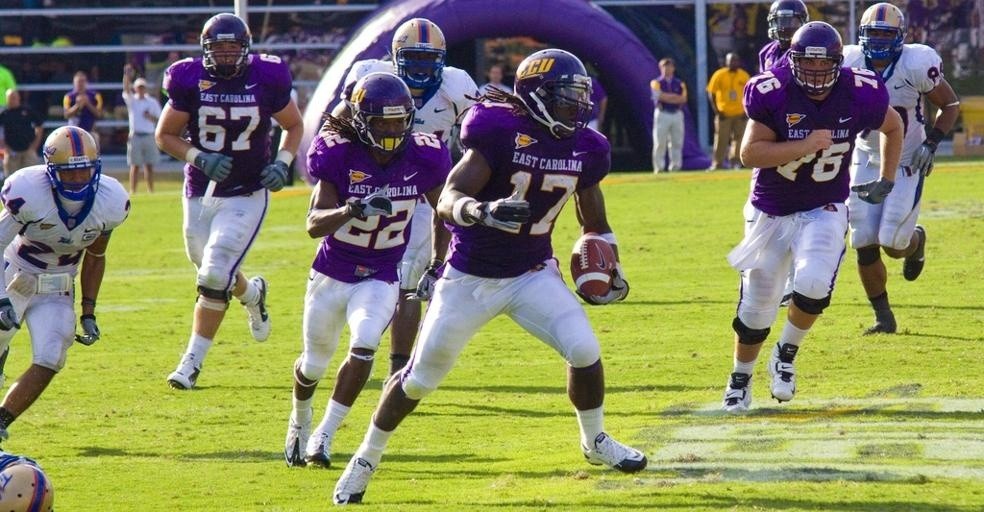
[767,0,843,96]
[0,464,53,512]
[515,49,595,141]
[346,18,446,156]
[858,3,905,58]
[43,126,100,200]
[201,13,251,78]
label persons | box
[154,12,304,389]
[0,126,131,441]
[1,0,961,196]
[721,0,960,417]
[283,17,648,502]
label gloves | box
[851,177,894,204]
[464,191,529,234]
[193,152,232,182]
[75,315,100,345]
[404,261,444,302]
[259,160,288,191]
[910,139,937,176]
[348,194,392,219]
[0,298,20,331]
[575,267,628,304]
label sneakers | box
[768,342,798,403]
[862,324,896,334]
[722,372,752,414]
[903,225,925,281]
[581,432,646,472]
[168,354,202,388]
[242,276,270,341]
[285,406,381,504]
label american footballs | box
[570,231,616,299]
